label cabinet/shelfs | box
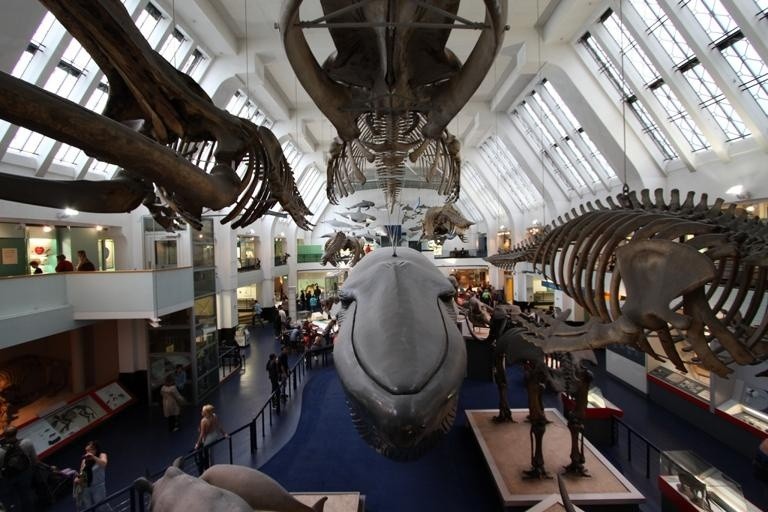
[647,365,710,426]
[561,387,623,451]
[714,398,768,460]
[656,448,762,511]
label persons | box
[30,262,42,274]
[278,345,292,398]
[76,250,95,272]
[194,404,228,467]
[172,363,187,390]
[289,326,301,349]
[55,255,74,272]
[300,285,323,311]
[73,441,112,511]
[161,374,188,432]
[266,353,282,407]
[252,300,266,328]
[277,305,289,329]
